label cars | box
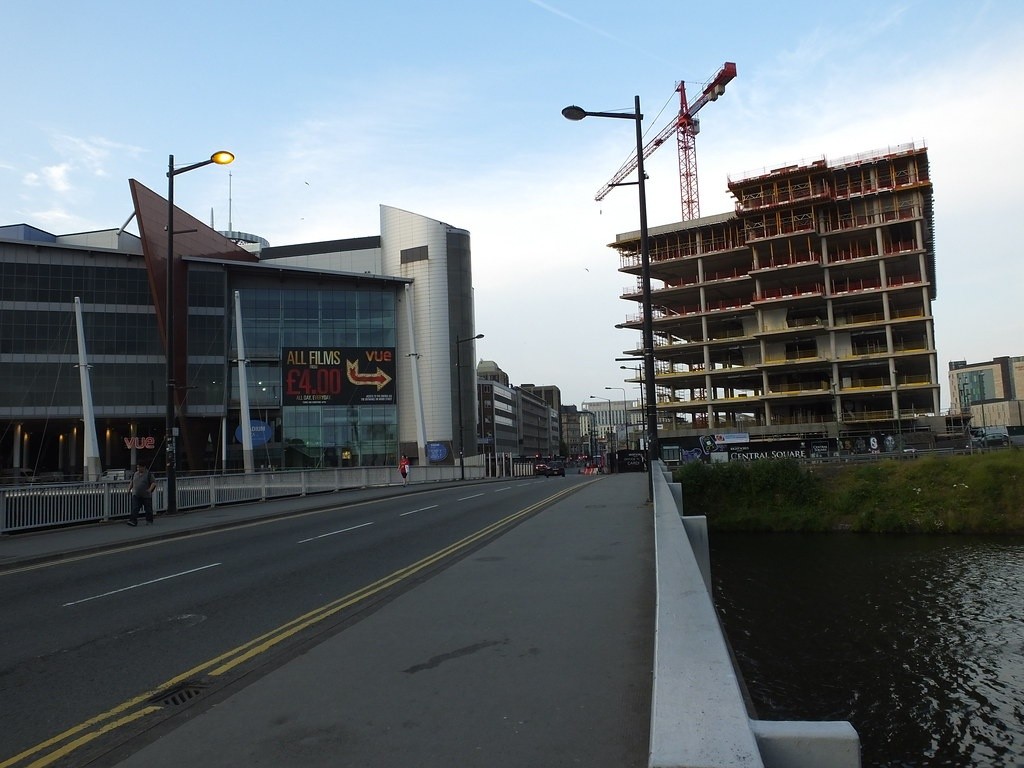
[965,432,1012,449]
[535,464,547,476]
[902,445,918,458]
[547,460,565,478]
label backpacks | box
[130,471,156,492]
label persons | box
[396,455,409,488]
[126,461,156,526]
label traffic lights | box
[505,454,509,463]
[534,453,542,461]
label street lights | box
[620,364,646,452]
[456,333,485,479]
[589,396,613,451]
[577,411,598,469]
[605,387,628,451]
[561,96,660,506]
[167,151,236,517]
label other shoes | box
[127,520,137,526]
[407,483,409,485]
[146,521,153,526]
[402,485,406,487]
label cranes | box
[593,61,739,226]
